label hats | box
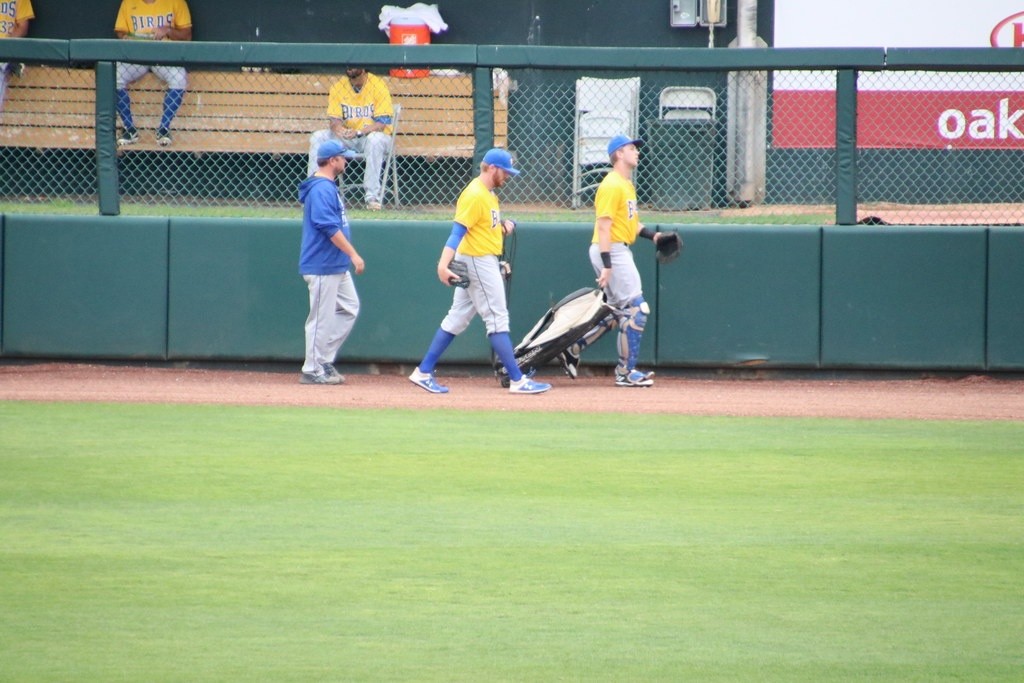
[482,149,520,174]
[607,135,644,156]
[317,140,355,159]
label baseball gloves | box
[436,256,472,290]
[655,231,685,265]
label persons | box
[306,66,395,214]
[0,1,35,103]
[559,134,662,388]
[114,0,191,147]
[409,148,553,394]
[298,143,366,385]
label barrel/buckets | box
[389,16,430,79]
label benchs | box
[0,56,476,163]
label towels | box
[430,68,461,75]
[377,2,449,39]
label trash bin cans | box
[390,17,431,79]
[645,119,715,210]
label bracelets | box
[356,130,363,139]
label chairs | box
[658,85,716,121]
[573,76,639,209]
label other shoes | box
[299,363,345,385]
[510,374,551,394]
[156,128,172,146]
[614,368,655,388]
[9,62,25,79]
[364,197,381,211]
[116,127,139,145]
[408,367,448,393]
[556,345,580,379]
